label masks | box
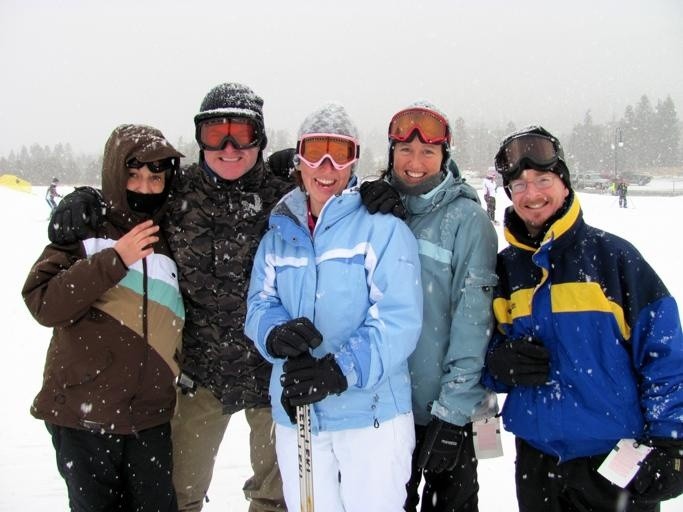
[127,190,165,212]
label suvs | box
[569,171,650,189]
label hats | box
[394,97,451,124]
[194,82,268,152]
[503,122,572,198]
[298,103,359,147]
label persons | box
[479,124,683,512]
[483,168,500,223]
[45,177,63,208]
[22,124,187,512]
[380,100,499,512]
[615,179,628,209]
[49,82,408,512]
[244,101,425,512]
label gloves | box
[279,353,347,425]
[418,418,469,476]
[266,317,323,360]
[632,438,682,506]
[487,333,550,388]
[359,175,408,219]
[47,186,105,247]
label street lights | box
[611,127,624,179]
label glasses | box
[387,107,451,146]
[494,133,560,175]
[295,132,360,171]
[195,118,264,152]
[128,159,175,173]
[502,175,556,195]
